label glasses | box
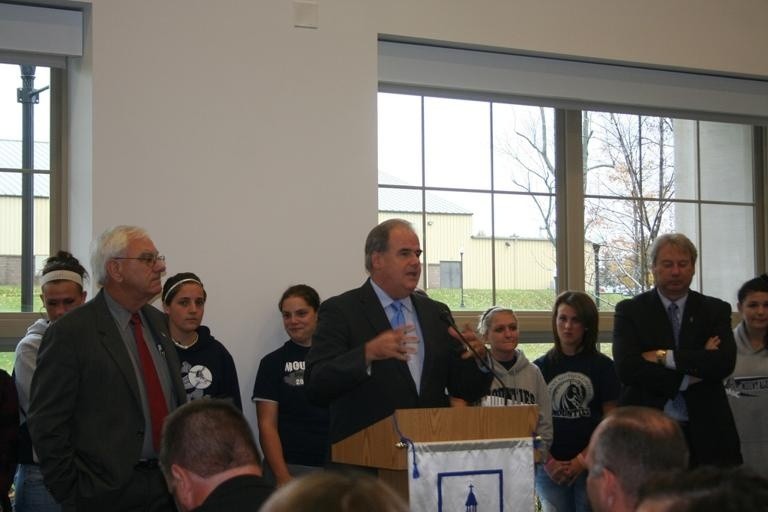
[113,255,166,267]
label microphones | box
[441,310,508,405]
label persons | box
[303,216,495,457]
[610,232,743,463]
[1,249,768,511]
[25,222,186,510]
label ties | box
[390,299,407,347]
[129,313,170,440]
[668,302,681,348]
